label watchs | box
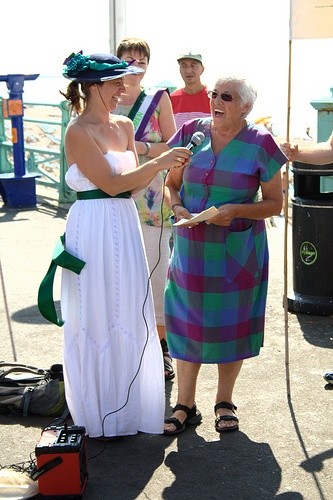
[143,142,152,156]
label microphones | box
[167,131,206,172]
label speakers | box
[34,425,90,497]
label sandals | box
[161,399,202,434]
[159,338,175,380]
[214,402,240,432]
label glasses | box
[212,92,242,102]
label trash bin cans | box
[283,161,333,316]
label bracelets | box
[172,204,182,211]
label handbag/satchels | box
[0,361,67,419]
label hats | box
[63,50,145,82]
[176,49,202,64]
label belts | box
[77,186,132,200]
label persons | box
[55,53,194,440]
[70,36,178,381]
[163,76,290,436]
[170,51,212,133]
[279,130,333,385]
